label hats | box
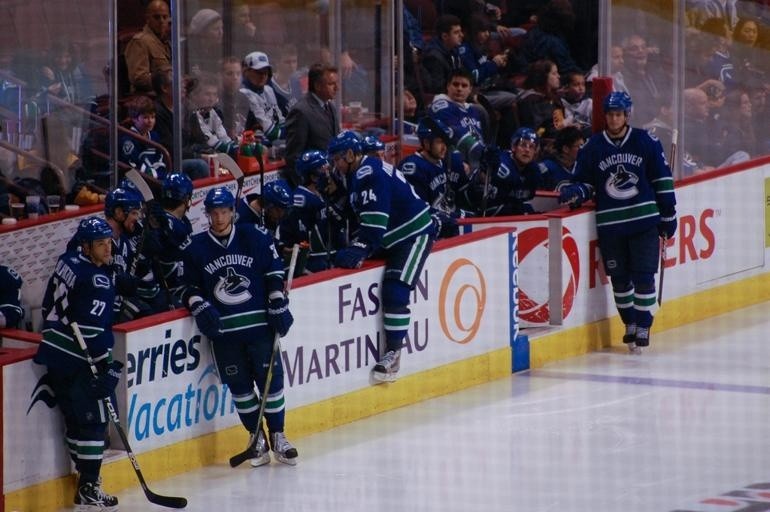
[190,8,222,36]
[242,50,274,71]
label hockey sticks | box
[217,152,244,212]
[231,243,301,468]
[647,130,678,320]
[252,147,265,227]
[324,93,497,269]
[71,321,189,509]
[119,166,154,324]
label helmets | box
[417,116,451,141]
[104,188,143,217]
[163,171,194,201]
[78,216,113,240]
[602,91,632,117]
[203,185,236,209]
[510,128,541,146]
[294,149,328,175]
[360,132,386,151]
[328,128,367,154]
[263,180,293,209]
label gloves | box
[659,216,677,240]
[266,298,294,340]
[558,185,590,210]
[334,243,374,271]
[90,360,126,401]
[190,300,224,342]
[115,272,142,299]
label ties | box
[324,103,334,133]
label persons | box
[1,2,770,372]
[33,216,124,506]
[173,186,299,459]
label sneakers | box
[622,322,636,343]
[635,324,650,346]
[372,348,400,374]
[72,481,119,506]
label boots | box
[246,429,270,460]
[268,430,298,460]
